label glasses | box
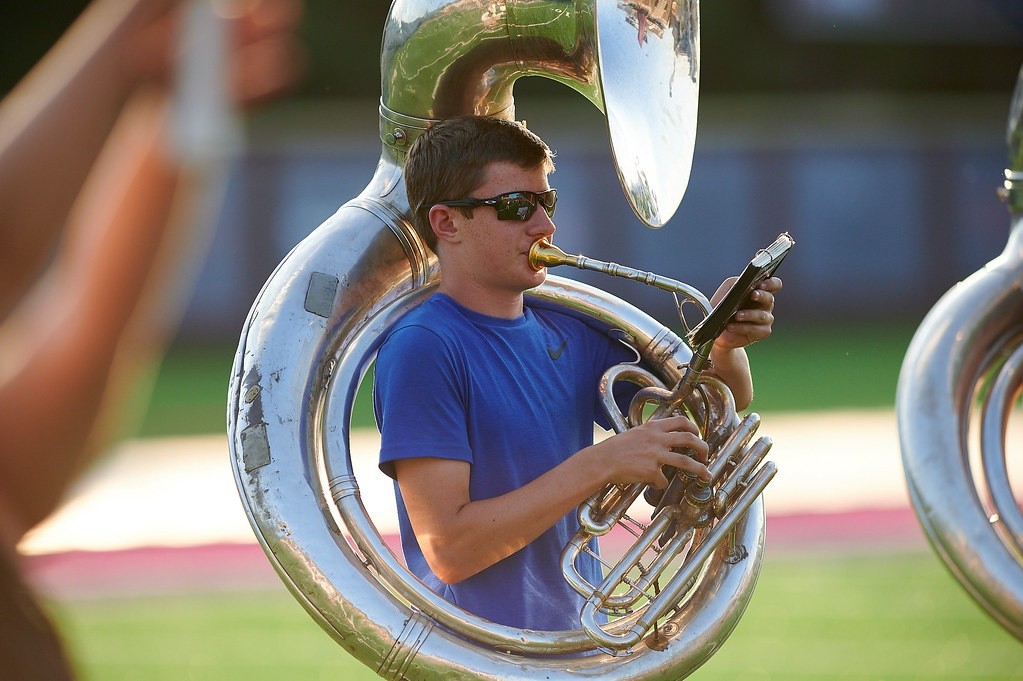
[423,187,559,222]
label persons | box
[0,0,300,681]
[374,115,781,661]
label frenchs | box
[889,59,1023,644]
[226,3,778,681]
